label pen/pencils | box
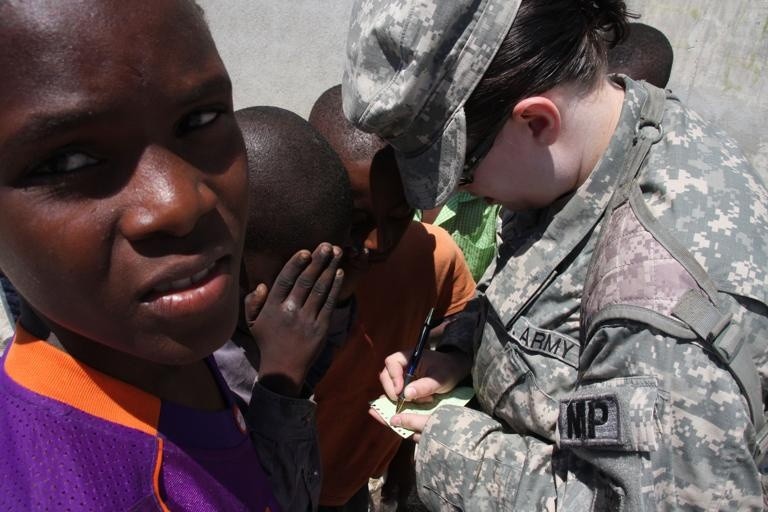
[396,308,436,413]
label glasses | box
[458,79,557,187]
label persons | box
[2,1,279,511]
[342,3,768,512]
[209,107,358,512]
[409,189,504,291]
[308,87,476,511]
[604,23,676,88]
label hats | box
[342,1,522,211]
